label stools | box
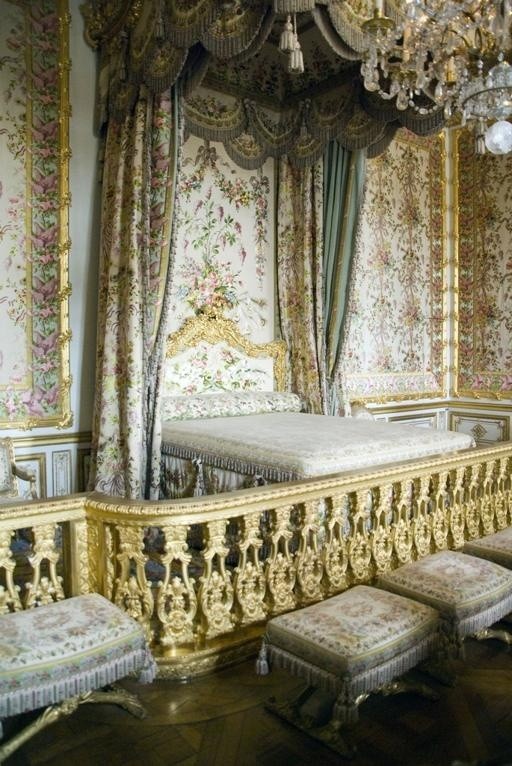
[255,585,450,765]
[375,546,512,684]
[0,590,161,766]
[464,522,511,571]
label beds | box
[161,303,476,563]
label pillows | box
[161,391,302,421]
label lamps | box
[354,0,512,155]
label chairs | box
[0,436,35,505]
